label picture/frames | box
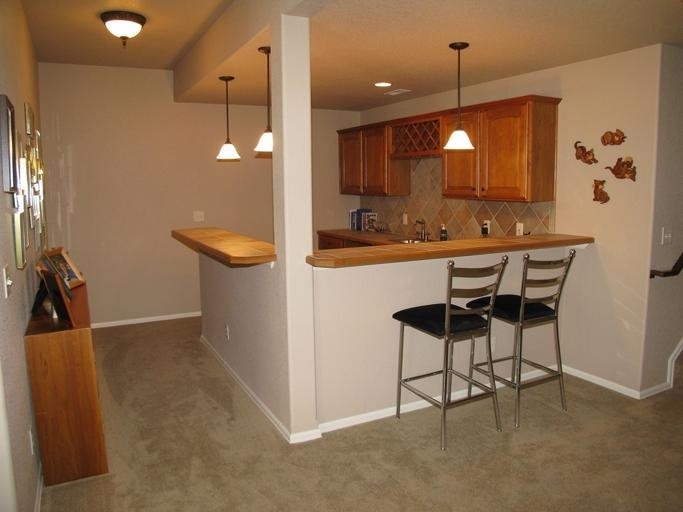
[1,92,84,329]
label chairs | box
[466,248,576,428]
[392,255,508,452]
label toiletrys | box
[440,224,447,241]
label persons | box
[59,262,76,281]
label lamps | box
[100,11,146,48]
[215,76,241,160]
[443,42,476,151]
[253,46,274,152]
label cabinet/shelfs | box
[441,94,560,203]
[337,122,411,196]
[24,272,109,488]
[318,235,371,250]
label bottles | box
[439,223,446,241]
[479,222,489,239]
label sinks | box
[388,238,424,243]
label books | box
[349,205,378,232]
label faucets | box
[415,219,426,240]
